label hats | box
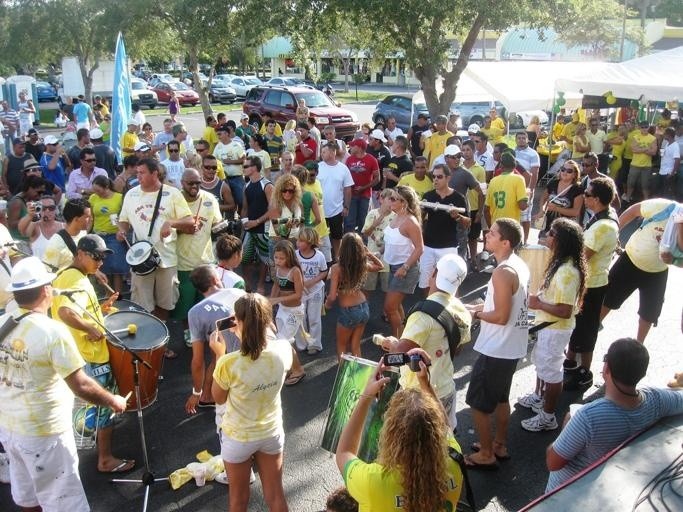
[90,128,103,139]
[217,113,249,133]
[78,234,114,254]
[127,120,138,126]
[7,256,57,292]
[24,159,44,170]
[133,142,150,152]
[436,254,467,296]
[13,128,58,145]
[347,129,387,149]
[443,123,481,156]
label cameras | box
[31,201,43,222]
[384,352,424,372]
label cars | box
[371,94,431,132]
[213,74,236,83]
[148,73,173,83]
[146,81,198,107]
[263,77,305,88]
[227,75,268,97]
[192,80,236,103]
[35,82,56,103]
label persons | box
[0,92,683,512]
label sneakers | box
[518,393,558,431]
[481,251,489,260]
[563,359,593,385]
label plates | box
[214,472,229,484]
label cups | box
[659,245,671,259]
[194,467,206,487]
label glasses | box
[169,149,217,186]
[86,251,105,260]
[85,159,95,163]
[433,154,461,178]
[549,230,557,238]
[244,164,255,168]
[36,190,56,211]
[391,196,404,202]
[282,188,295,193]
[560,162,597,197]
[31,168,42,171]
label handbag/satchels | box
[535,215,547,231]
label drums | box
[319,353,400,464]
[100,312,169,411]
[126,242,159,274]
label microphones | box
[0,240,21,249]
[52,288,85,297]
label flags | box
[110,31,132,166]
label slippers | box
[284,373,306,386]
[463,442,511,469]
[198,401,215,407]
[96,459,136,474]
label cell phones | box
[215,316,237,332]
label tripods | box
[66,299,170,512]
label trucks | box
[56,55,130,111]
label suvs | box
[179,72,206,82]
[130,80,157,110]
[242,86,360,144]
[450,101,507,129]
